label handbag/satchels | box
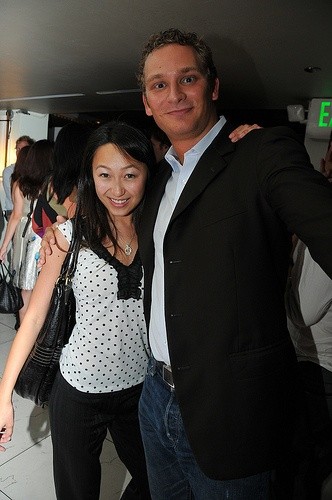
[0,260,24,313]
[15,279,77,409]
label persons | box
[150,123,172,163]
[0,118,90,333]
[278,126,332,500]
[36,26,332,500]
[0,119,265,499]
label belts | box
[152,358,175,388]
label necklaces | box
[108,224,137,257]
